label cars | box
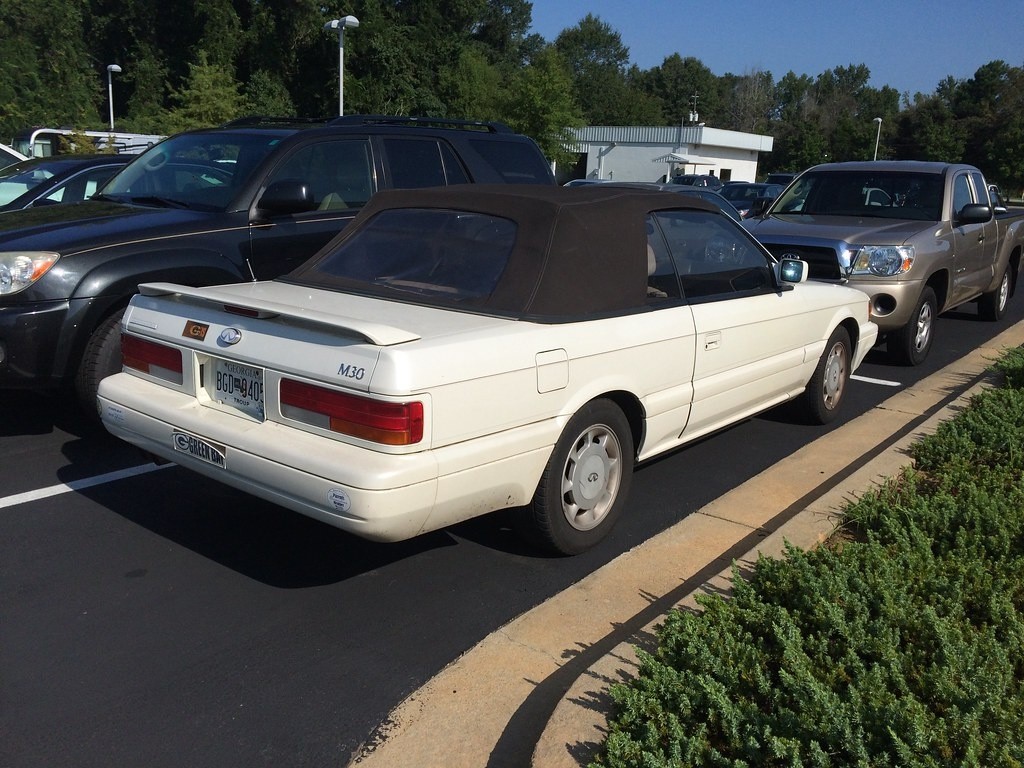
[0,142,97,207]
[715,183,784,220]
[0,153,235,213]
[200,159,238,186]
[663,174,721,191]
[562,179,611,186]
[95,184,880,556]
[580,182,744,223]
[763,173,799,185]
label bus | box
[11,127,148,159]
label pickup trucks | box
[738,160,1024,366]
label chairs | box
[924,189,944,207]
[647,243,668,298]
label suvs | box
[0,115,556,460]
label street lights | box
[323,15,360,117]
[871,117,883,162]
[106,64,122,130]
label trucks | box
[94,138,160,154]
